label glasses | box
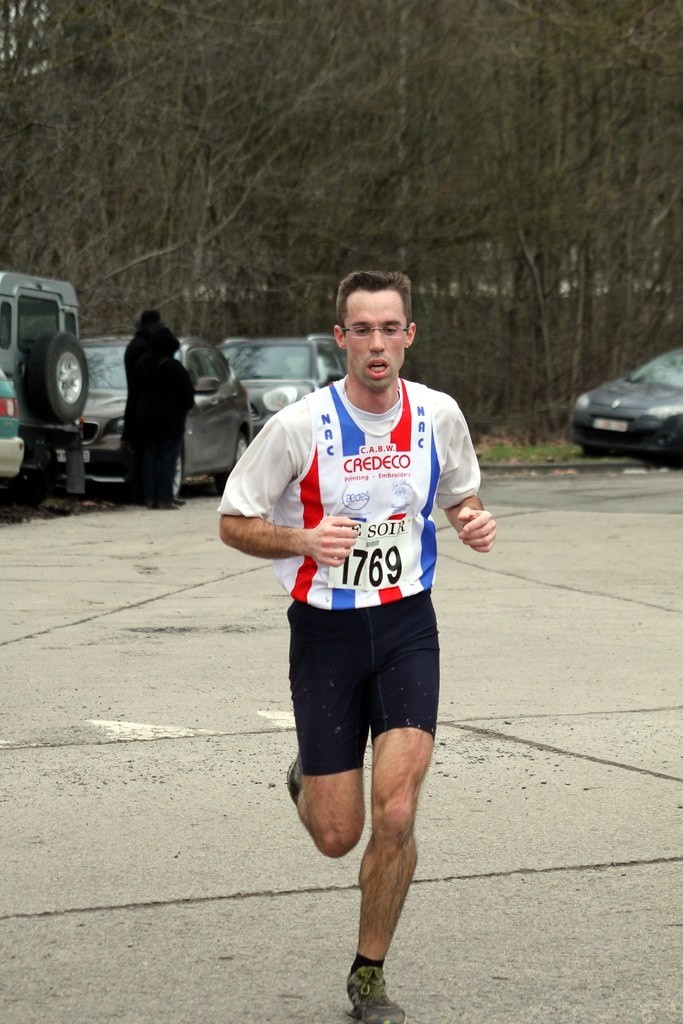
[340,323,410,338]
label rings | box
[487,534,492,541]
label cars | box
[570,348,682,469]
[74,334,254,501]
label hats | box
[134,310,165,331]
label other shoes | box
[151,501,179,510]
[172,499,186,506]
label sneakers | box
[286,753,305,807]
[346,967,407,1024]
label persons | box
[121,310,196,510]
[217,272,499,1024]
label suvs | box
[0,273,92,508]
[218,335,350,440]
